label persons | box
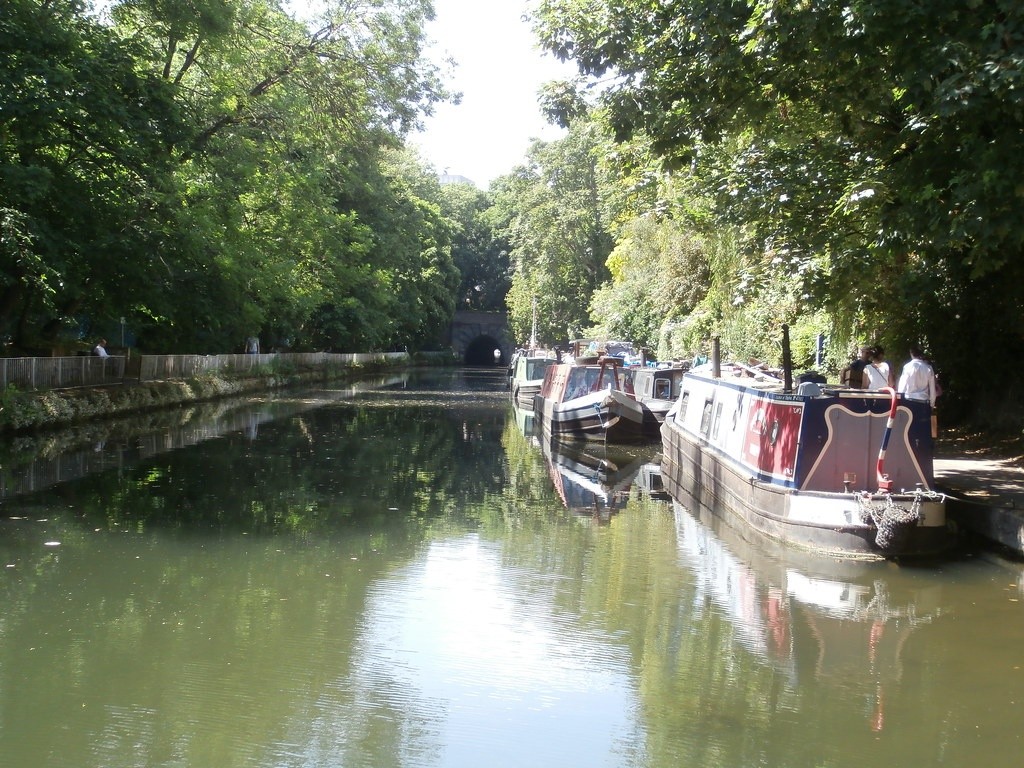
[897,346,937,449]
[861,345,895,391]
[839,346,873,389]
[93,339,114,357]
[244,331,260,354]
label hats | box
[859,346,871,353]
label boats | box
[661,325,955,561]
[531,351,647,445]
[506,295,695,432]
